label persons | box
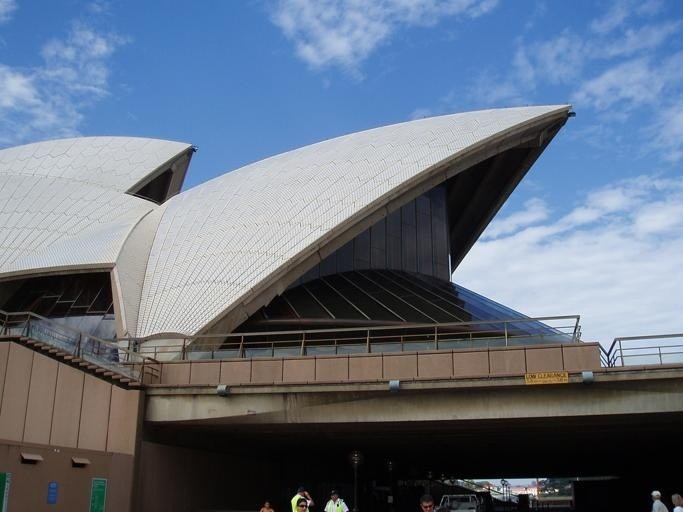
[651,490,668,512]
[671,493,683,512]
[292,497,309,512]
[290,485,314,512]
[419,494,436,511]
[324,490,349,511]
[260,501,274,511]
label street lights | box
[426,470,455,494]
[351,450,360,512]
[501,479,510,501]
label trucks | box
[437,492,482,512]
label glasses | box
[297,505,307,508]
[421,504,433,509]
